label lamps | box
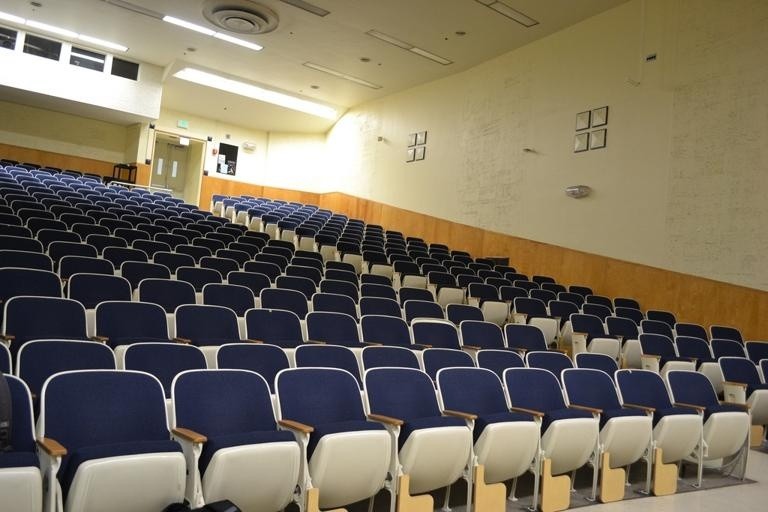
[203,0,279,34]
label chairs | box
[0,159,171,275]
[322,207,456,279]
[172,185,323,273]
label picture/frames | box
[574,106,608,152]
[405,130,427,162]
